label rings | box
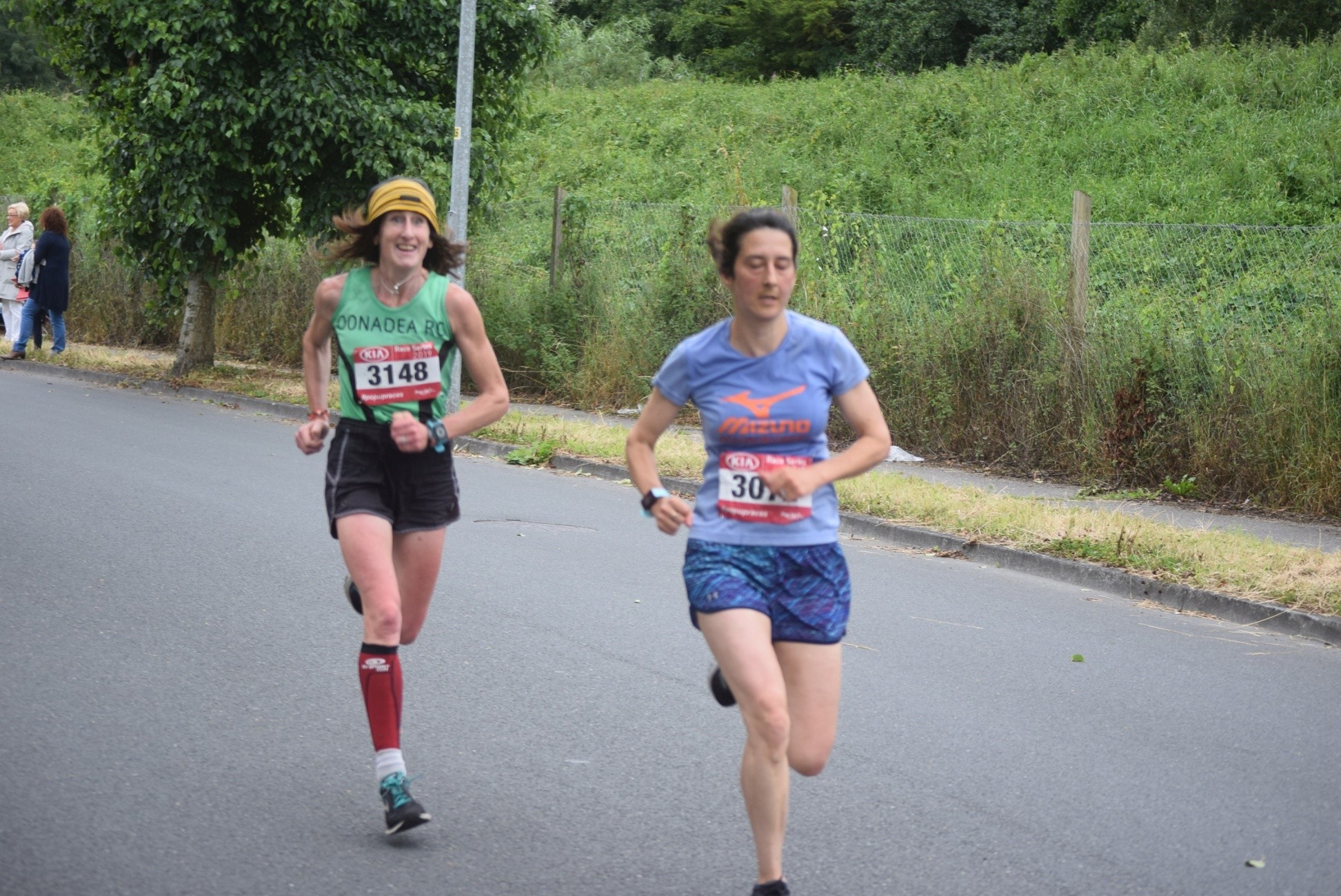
[401,436,405,445]
[779,489,786,498]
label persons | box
[626,208,890,896]
[295,178,510,844]
[0,201,72,360]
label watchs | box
[641,487,669,518]
[427,418,448,453]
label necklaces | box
[380,272,411,295]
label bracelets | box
[427,428,431,450]
[307,409,330,422]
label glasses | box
[6,214,19,217]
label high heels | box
[2,350,26,360]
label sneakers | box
[751,876,790,896]
[379,773,429,835]
[710,668,738,708]
[342,575,363,615]
